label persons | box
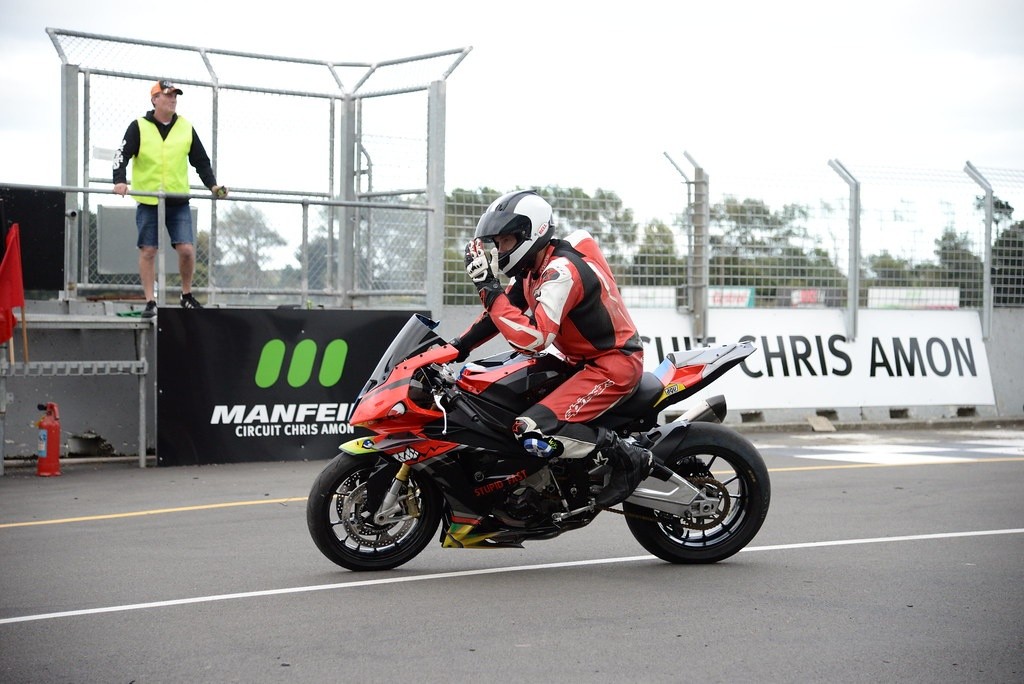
[112,80,228,318]
[447,190,653,508]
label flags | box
[0,224,24,345]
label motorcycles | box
[307,313,771,571]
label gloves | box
[462,238,505,306]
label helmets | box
[474,190,556,278]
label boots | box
[594,431,654,508]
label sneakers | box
[142,301,158,317]
[179,294,204,308]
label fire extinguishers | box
[36,402,61,477]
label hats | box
[150,79,183,98]
